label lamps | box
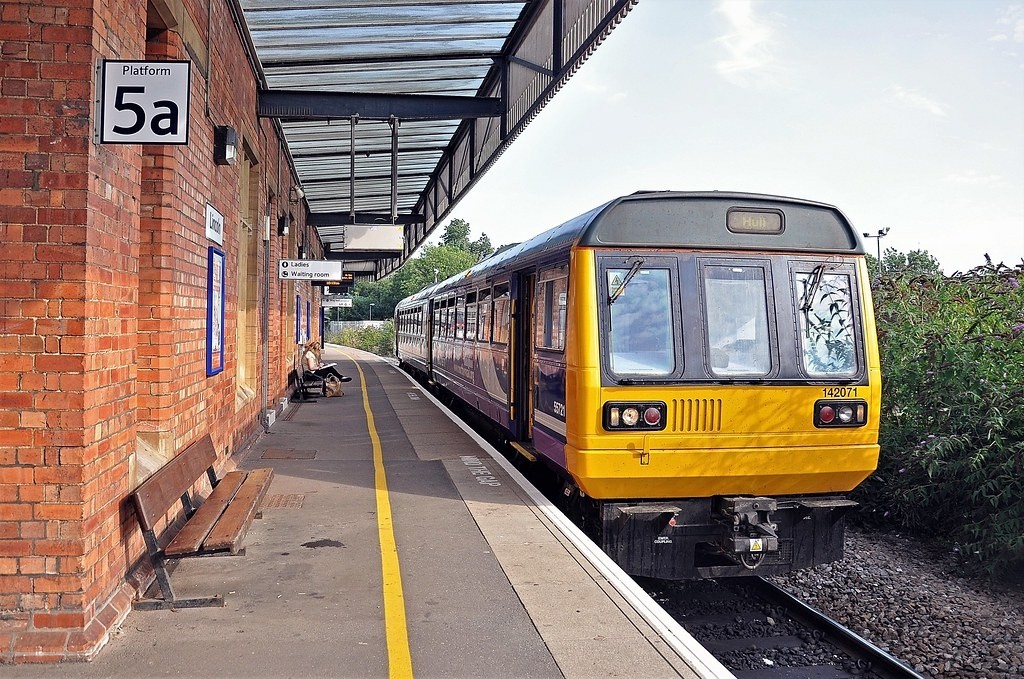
[289,184,305,203]
[213,125,239,166]
[299,246,307,259]
[279,216,290,238]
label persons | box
[301,342,352,382]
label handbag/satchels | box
[321,373,344,397]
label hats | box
[305,341,314,348]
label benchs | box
[128,432,273,611]
[290,365,322,403]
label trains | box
[394,190,880,583]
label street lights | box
[864,225,890,272]
[370,304,375,321]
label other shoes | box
[339,376,353,382]
